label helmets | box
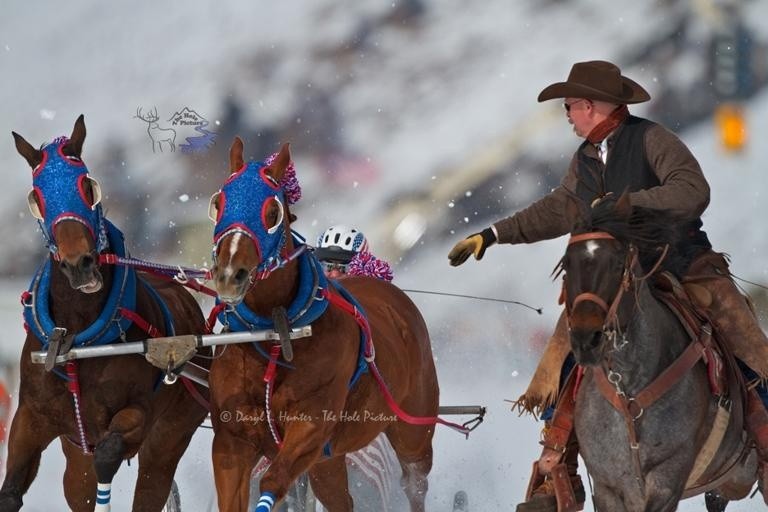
[314,226,368,262]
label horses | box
[547,183,768,512]
[0,114,214,512]
[207,135,440,512]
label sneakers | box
[517,476,584,512]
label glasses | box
[564,98,582,111]
[321,261,349,273]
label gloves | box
[448,229,496,266]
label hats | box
[538,61,650,104]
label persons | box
[446,56,766,512]
[315,223,393,280]
